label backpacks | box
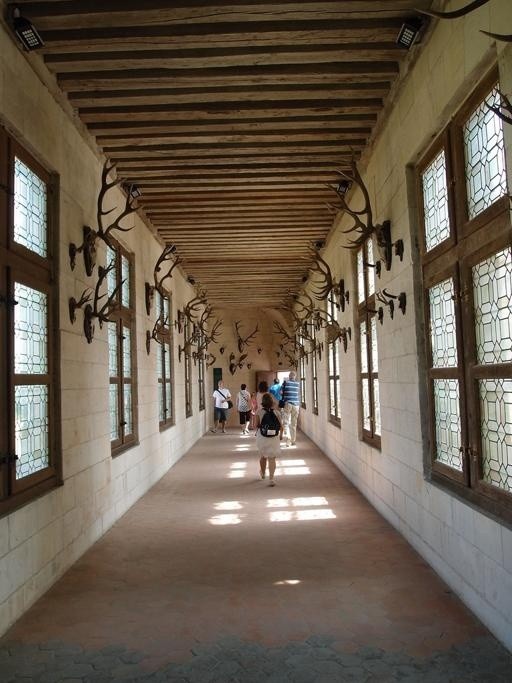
[260,407,281,436]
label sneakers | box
[242,428,249,434]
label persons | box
[210,371,300,447]
[254,393,284,487]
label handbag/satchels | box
[228,400,233,409]
